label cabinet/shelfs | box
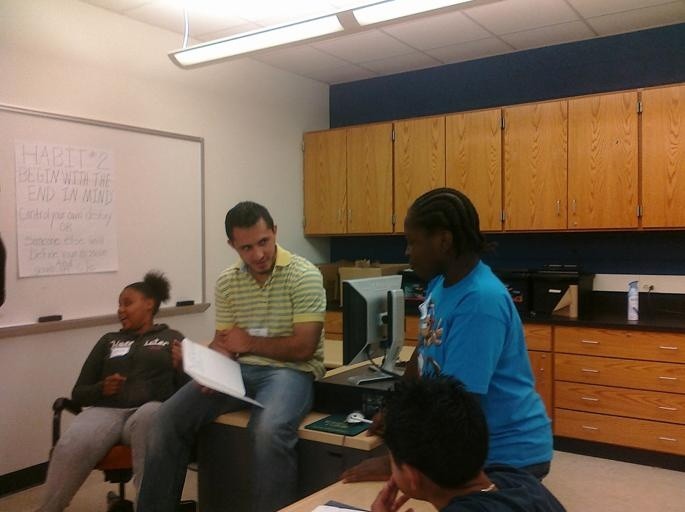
[555,325,685,457]
[323,311,420,345]
[520,324,554,441]
[393,106,502,236]
[301,119,393,237]
[502,89,639,231]
[639,82,684,230]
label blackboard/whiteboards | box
[0,105,212,338]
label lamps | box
[167,1,503,71]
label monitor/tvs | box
[341,274,405,385]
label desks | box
[205,338,438,512]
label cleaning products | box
[627,280,640,321]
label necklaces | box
[480,483,496,492]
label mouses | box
[346,412,364,423]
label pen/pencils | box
[351,417,374,424]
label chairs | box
[46,391,198,511]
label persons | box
[370,370,567,512]
[35,270,185,512]
[137,202,327,512]
[340,186,554,484]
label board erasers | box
[176,300,194,307]
[39,315,62,322]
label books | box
[180,338,264,410]
